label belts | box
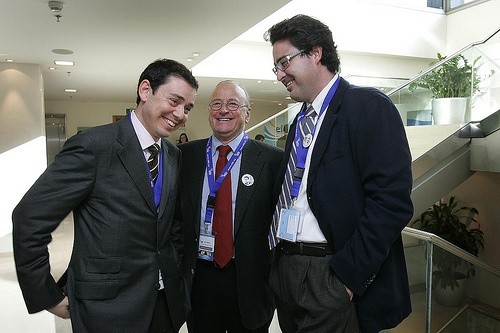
[275,239,334,257]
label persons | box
[178,133,189,143]
[254,133,266,142]
[262,15,415,333]
[174,80,284,333]
[276,123,289,148]
[10,60,201,333]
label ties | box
[212,145,234,270]
[269,104,317,251]
[145,143,160,189]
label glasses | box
[271,45,307,76]
[208,101,250,110]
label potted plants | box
[409,54,482,126]
[409,197,485,306]
